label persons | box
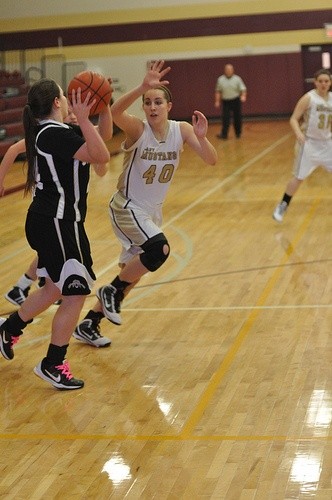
[272,69,332,222]
[0,77,114,390]
[0,105,108,306]
[73,58,217,347]
[214,64,247,139]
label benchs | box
[0,69,31,164]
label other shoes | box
[272,201,288,223]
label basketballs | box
[67,70,112,117]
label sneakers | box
[33,357,84,390]
[71,317,112,347]
[35,279,60,304]
[0,318,24,360]
[95,284,125,326]
[4,286,31,306]
